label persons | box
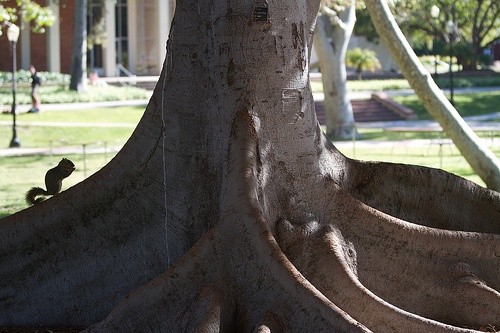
[29,65,42,111]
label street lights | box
[430,4,440,86]
[7,24,21,148]
[446,19,456,107]
